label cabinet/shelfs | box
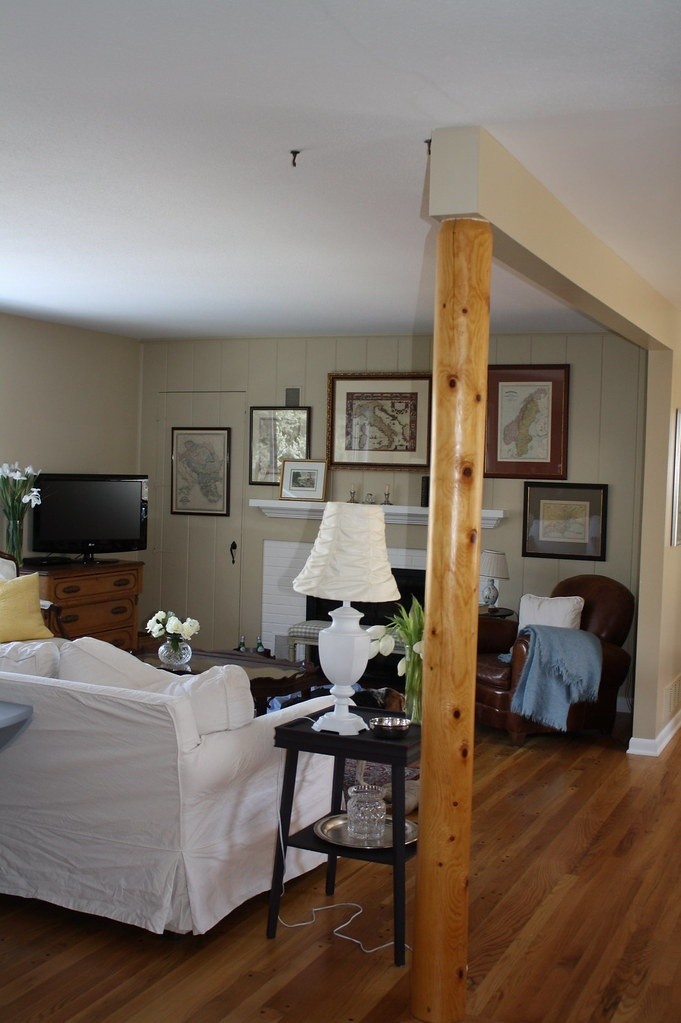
[18,557,145,653]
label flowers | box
[145,610,200,653]
[365,595,425,725]
[0,461,42,568]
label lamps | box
[293,501,402,737]
[480,549,509,605]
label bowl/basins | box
[369,717,411,738]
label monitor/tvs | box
[33,474,148,565]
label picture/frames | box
[249,406,311,487]
[326,371,432,474]
[671,409,681,546]
[171,426,231,517]
[483,363,569,479]
[278,459,328,501]
[521,480,608,562]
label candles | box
[385,485,389,493]
[351,484,354,491]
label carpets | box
[342,757,421,803]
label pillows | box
[0,572,54,643]
[516,594,585,638]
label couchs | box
[0,638,355,945]
[475,574,635,748]
[0,552,54,631]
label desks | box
[290,620,408,663]
[267,703,421,967]
[0,701,34,752]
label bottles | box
[256,636,264,656]
[239,636,246,652]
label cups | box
[346,785,386,840]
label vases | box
[5,521,23,569]
[405,643,423,726]
[158,635,192,664]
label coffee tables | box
[477,607,513,619]
[127,647,316,716]
